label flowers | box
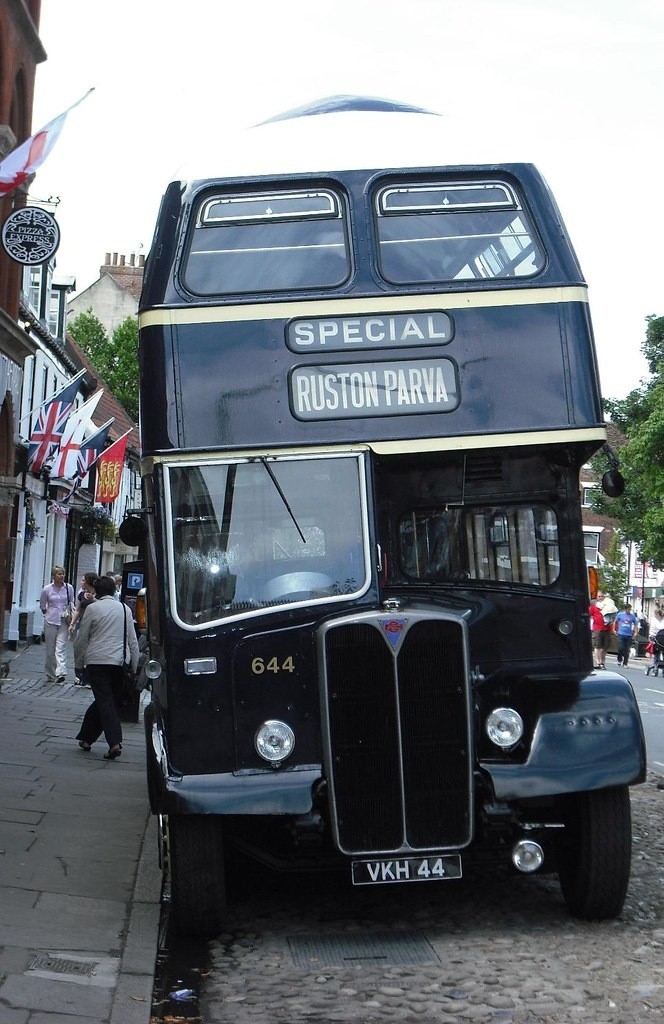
[92,504,108,517]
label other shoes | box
[609,623,614,626]
[624,664,627,668]
[594,665,602,669]
[55,675,65,683]
[46,675,53,681]
[600,665,606,670]
[617,662,622,668]
[604,622,609,626]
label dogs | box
[0,662,10,694]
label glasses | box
[598,596,603,598]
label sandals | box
[104,741,122,758]
[79,740,91,751]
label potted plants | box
[78,508,116,547]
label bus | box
[113,159,648,934]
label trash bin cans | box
[634,635,651,657]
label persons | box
[589,593,664,673]
[38,565,142,758]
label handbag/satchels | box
[646,641,653,653]
[121,660,140,706]
[120,672,140,723]
[64,602,76,626]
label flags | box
[0,112,67,200]
[11,370,131,507]
